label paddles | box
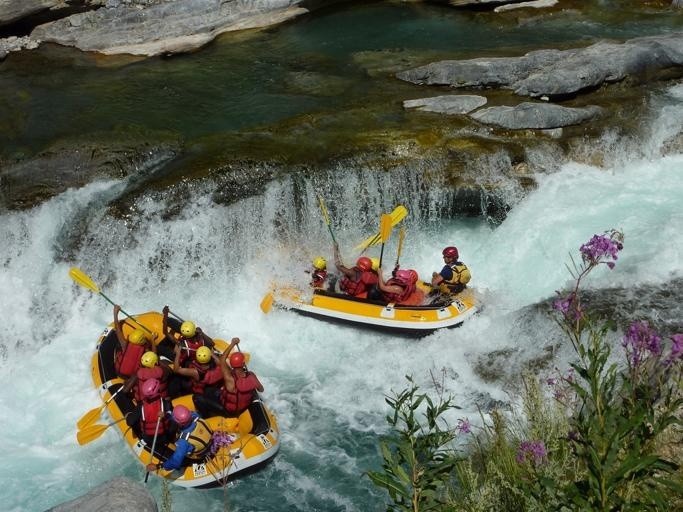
[76,411,132,447]
[318,198,343,262]
[181,346,250,363]
[260,286,277,315]
[354,205,407,269]
[75,384,126,430]
[166,310,234,355]
[68,268,154,335]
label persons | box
[310,256,419,302]
[113,304,265,471]
[424,246,472,305]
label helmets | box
[357,257,380,271]
[180,321,195,337]
[395,270,410,284]
[196,345,212,364]
[172,404,191,427]
[142,378,160,400]
[313,257,326,270]
[129,329,158,368]
[443,247,458,259]
[230,352,245,368]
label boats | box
[273,280,486,340]
[91,311,281,489]
